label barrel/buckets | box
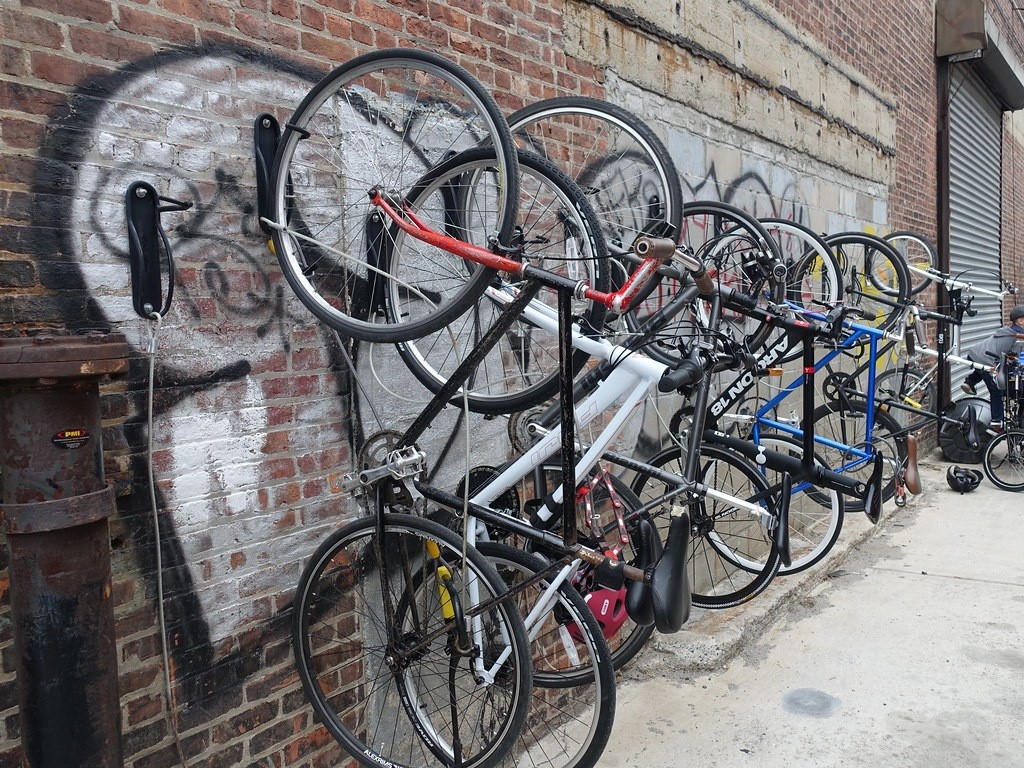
[941,397,995,465]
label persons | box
[962,307,1024,436]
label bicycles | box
[255,45,1024,768]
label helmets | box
[947,465,984,491]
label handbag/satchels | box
[992,352,1006,390]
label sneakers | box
[986,424,1006,441]
[961,379,978,395]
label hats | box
[1010,305,1024,320]
